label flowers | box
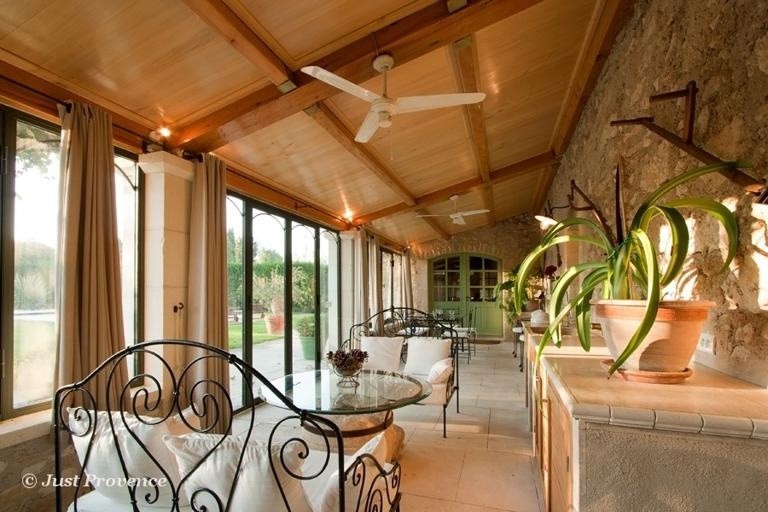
[327,347,368,374]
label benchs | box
[344,308,458,440]
[51,339,406,512]
[252,304,266,318]
[228,307,239,322]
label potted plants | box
[496,264,538,312]
[297,316,317,360]
[494,159,756,383]
[236,265,315,337]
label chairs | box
[394,304,479,365]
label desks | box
[256,368,434,466]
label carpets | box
[468,339,501,344]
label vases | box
[334,364,363,387]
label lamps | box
[533,202,572,231]
[148,124,171,143]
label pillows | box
[161,431,314,512]
[315,432,389,512]
[403,337,452,377]
[64,400,207,508]
[427,359,453,382]
[360,336,404,370]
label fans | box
[415,194,492,226]
[299,54,487,146]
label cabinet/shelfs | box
[512,318,768,511]
